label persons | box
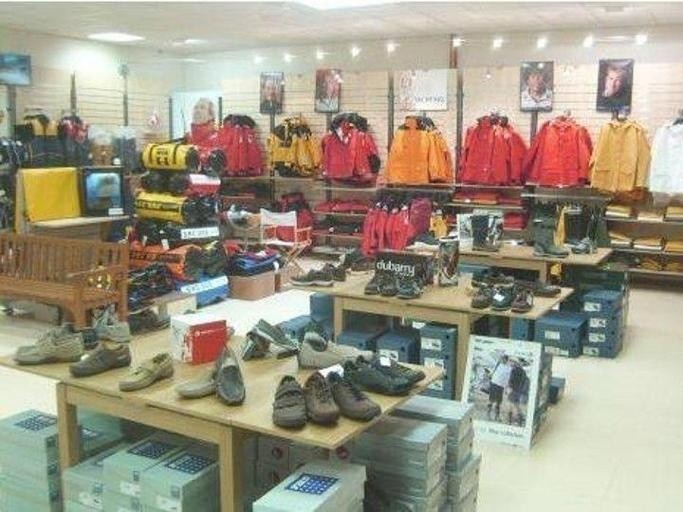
[260,79,281,113]
[482,354,512,421]
[182,97,220,166]
[598,65,630,108]
[521,68,552,108]
[505,355,532,424]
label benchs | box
[0,231,130,334]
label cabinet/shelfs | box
[0,318,449,512]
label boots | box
[532,221,569,258]
[470,215,498,252]
[243,319,298,360]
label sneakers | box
[273,371,382,429]
[13,321,95,366]
[364,256,434,299]
[299,332,374,369]
[127,307,171,335]
[69,342,131,378]
[175,345,245,406]
[471,270,561,312]
[571,236,597,254]
[94,311,133,343]
[119,353,175,391]
[288,263,345,287]
[338,249,376,276]
[343,352,426,396]
